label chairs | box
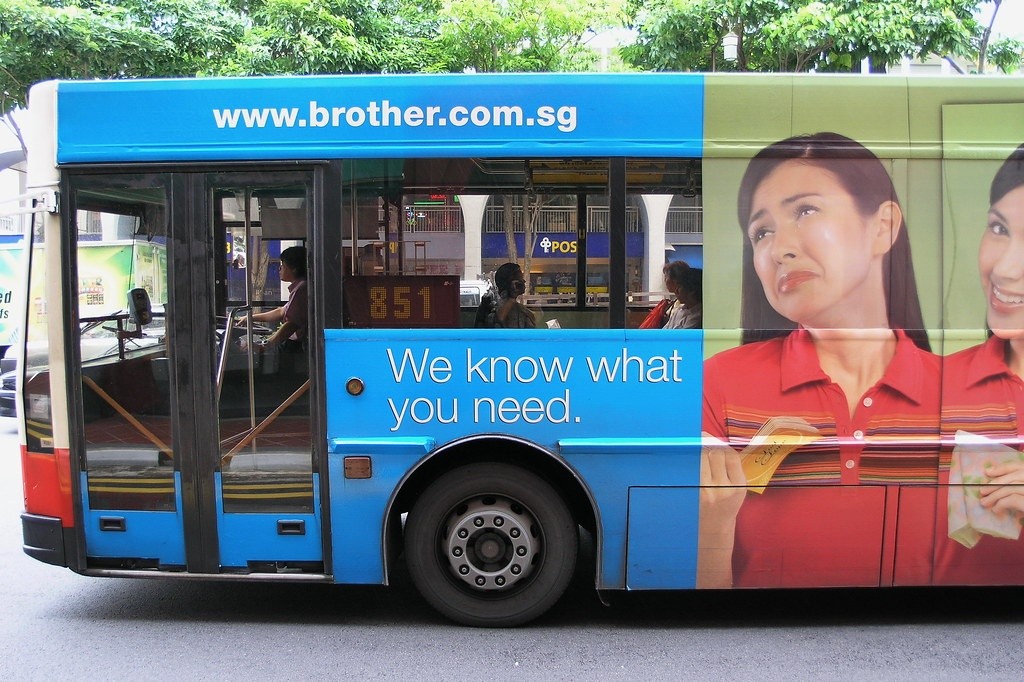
[473,294,494,329]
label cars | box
[0,303,167,417]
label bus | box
[16,72,1023,630]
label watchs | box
[262,340,271,349]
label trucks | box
[0,239,168,349]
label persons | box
[662,268,703,330]
[696,130,944,589]
[493,262,536,329]
[931,140,1023,587]
[237,245,309,401]
[656,260,690,330]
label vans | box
[459,280,489,307]
